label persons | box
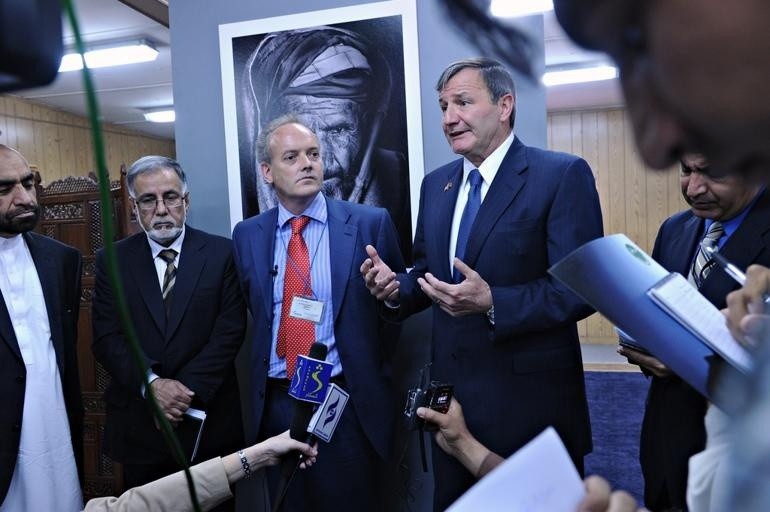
[84,428,317,510]
[233,114,407,509]
[416,393,649,512]
[1,145,83,510]
[357,61,603,510]
[550,0,770,511]
[239,26,408,263]
[615,152,769,510]
[90,154,250,511]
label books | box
[138,406,205,470]
[548,231,761,420]
[613,328,651,356]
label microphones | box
[289,342,330,442]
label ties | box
[276,218,315,380]
[688,222,725,288]
[158,249,179,320]
[453,169,483,284]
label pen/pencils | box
[704,245,770,305]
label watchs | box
[486,306,494,324]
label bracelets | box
[236,448,253,480]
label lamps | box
[61,38,160,72]
[144,106,176,126]
[543,61,619,89]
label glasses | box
[136,194,188,209]
